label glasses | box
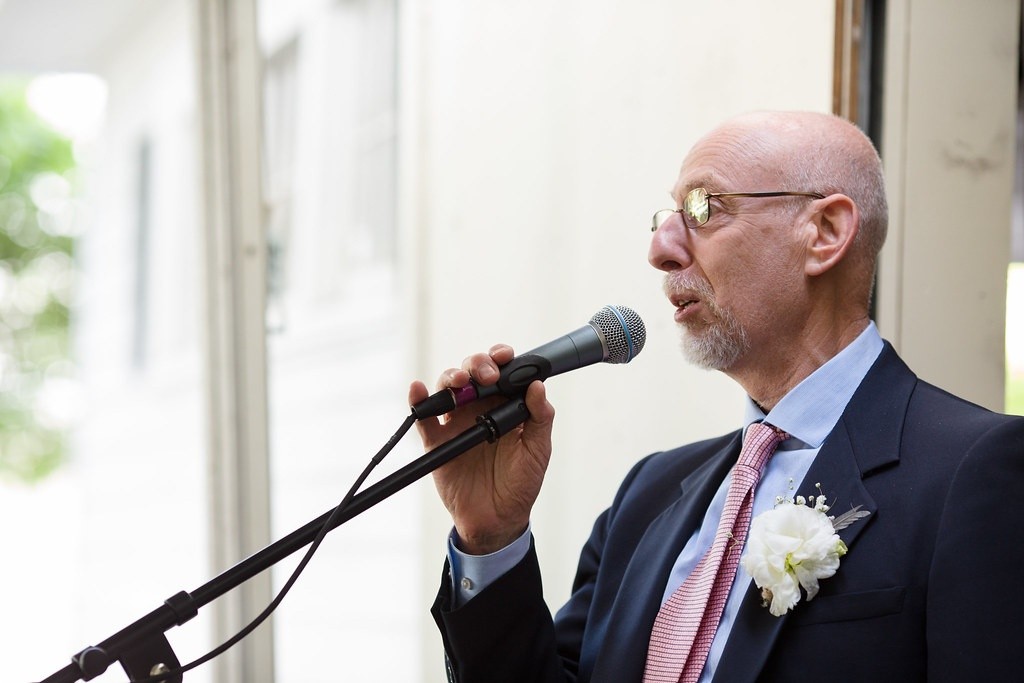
[649,188,830,234]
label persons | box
[408,112,1024,683]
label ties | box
[646,414,793,682]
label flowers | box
[724,476,871,616]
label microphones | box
[411,305,646,422]
